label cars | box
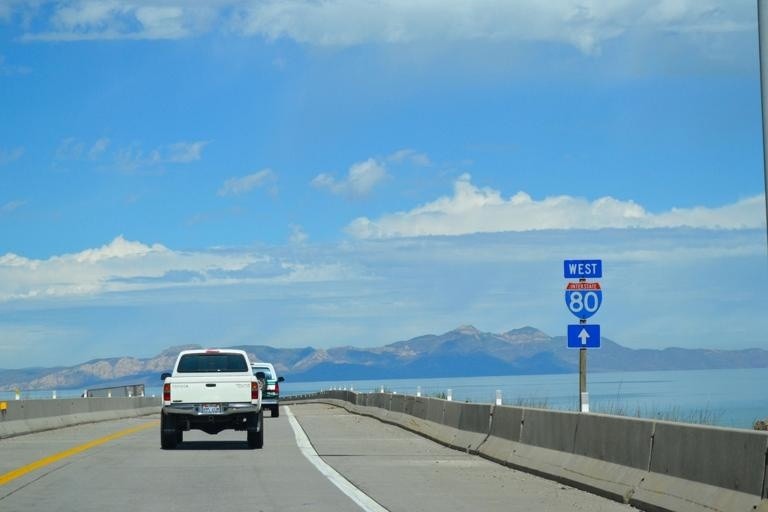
[161,349,285,448]
